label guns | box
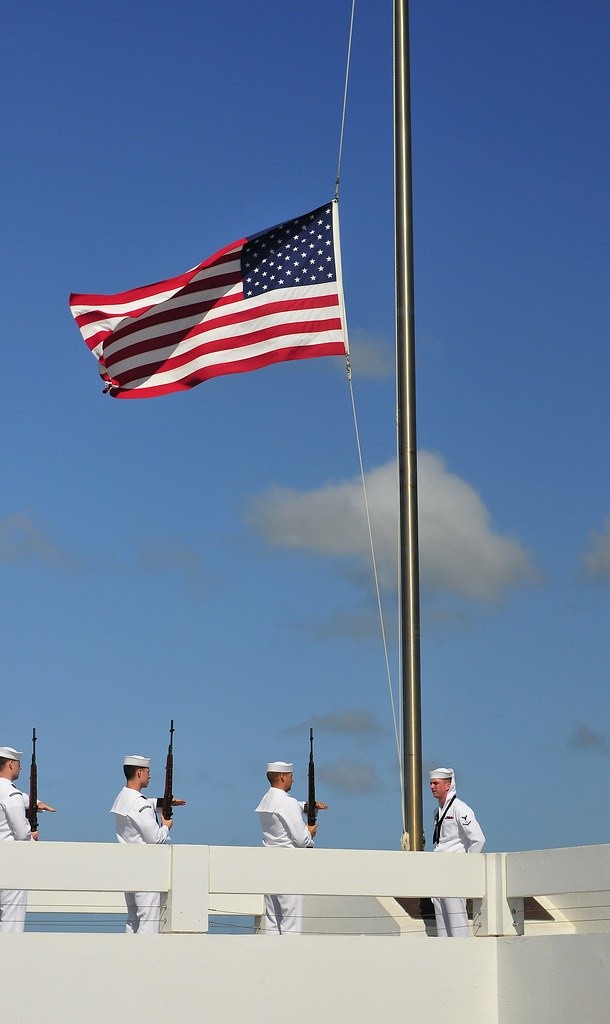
[303,728,317,849]
[25,727,38,841]
[161,719,174,832]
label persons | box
[109,756,186,934]
[0,747,56,933]
[430,767,486,937]
[254,761,330,936]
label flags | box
[69,201,347,399]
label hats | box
[0,747,23,761]
[124,755,151,767]
[267,761,293,772]
[430,767,455,779]
